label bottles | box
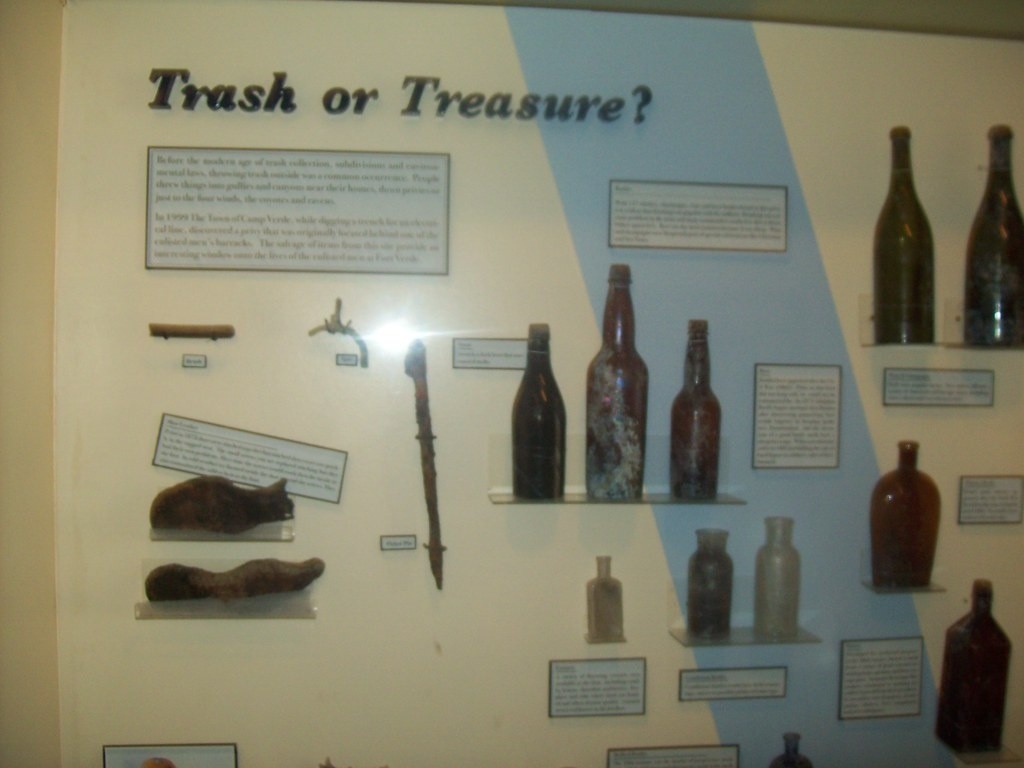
[768,733,813,768]
[964,125,1024,348]
[512,323,566,500]
[586,556,623,641]
[872,126,934,345]
[754,516,802,642]
[870,441,941,590]
[686,527,734,641]
[935,578,1012,753]
[586,264,649,501]
[670,318,721,500]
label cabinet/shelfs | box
[131,520,316,620]
[861,339,1024,596]
[490,490,824,646]
